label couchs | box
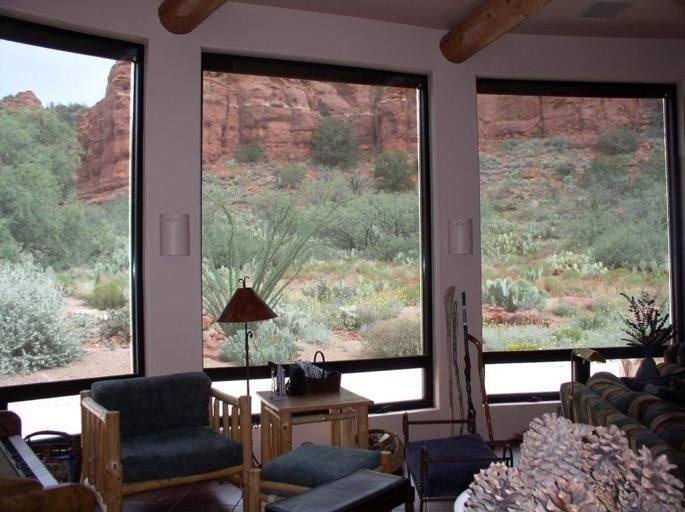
[557,371,685,495]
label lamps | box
[214,274,277,397]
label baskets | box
[285,351,341,394]
[11,430,81,481]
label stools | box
[249,411,514,512]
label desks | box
[257,386,376,464]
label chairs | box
[0,371,252,512]
